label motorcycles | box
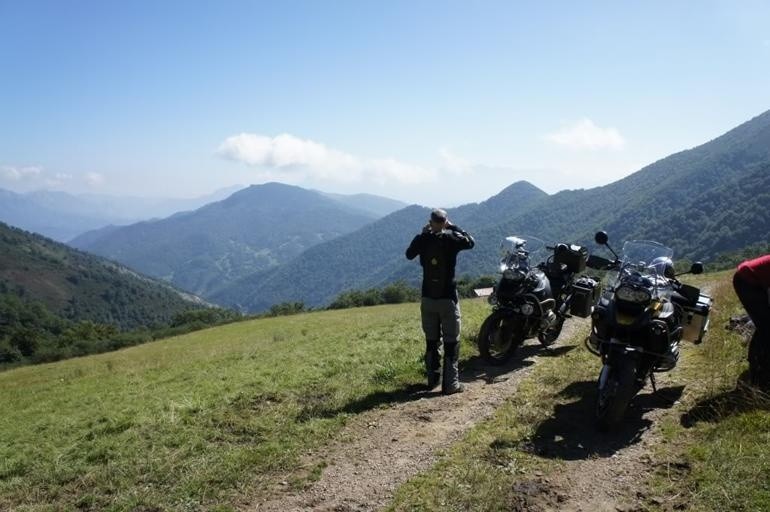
[474,233,602,366]
[569,230,713,426]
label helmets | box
[648,256,675,279]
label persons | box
[406,209,476,395]
[732,253,770,392]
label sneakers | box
[456,383,464,393]
[428,381,441,391]
